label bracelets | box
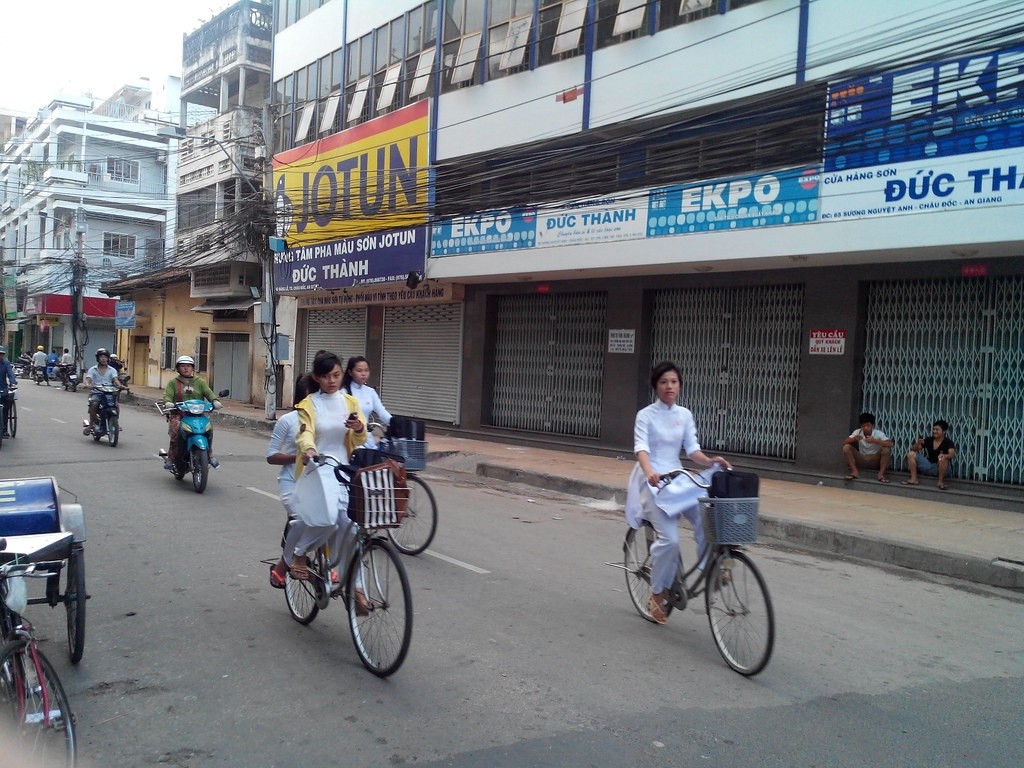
[291,454,296,463]
[354,423,365,433]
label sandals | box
[650,591,667,625]
[290,555,309,579]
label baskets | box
[387,441,428,471]
[698,497,760,545]
[342,485,411,531]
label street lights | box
[38,210,84,382]
[157,126,277,420]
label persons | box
[841,412,893,484]
[266,372,340,588]
[902,420,957,491]
[289,350,371,613]
[341,355,393,450]
[164,356,223,471]
[625,362,735,624]
[0,345,134,436]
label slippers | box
[332,571,339,584]
[901,480,919,485]
[878,476,890,483]
[269,564,286,588]
[937,482,949,490]
[845,474,860,480]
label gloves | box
[165,402,174,409]
[213,400,223,410]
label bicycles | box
[368,422,439,555]
[261,453,413,677]
[605,466,775,676]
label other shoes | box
[83,428,90,436]
[165,460,173,469]
[210,458,219,468]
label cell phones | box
[345,412,357,426]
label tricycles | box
[0,476,91,768]
[0,385,17,447]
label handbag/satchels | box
[389,417,426,441]
[710,471,761,496]
[333,448,410,527]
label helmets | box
[177,356,195,365]
[95,348,109,357]
[38,346,44,350]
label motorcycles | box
[154,389,230,493]
[83,384,122,447]
[8,353,77,392]
